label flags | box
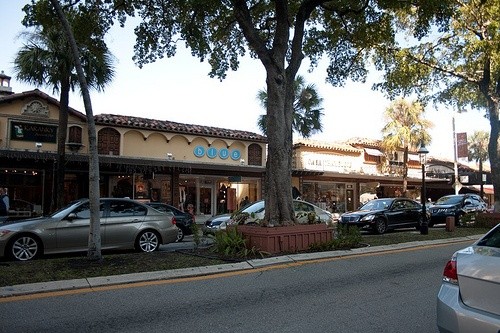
[457,132,468,158]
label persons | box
[0,187,10,216]
[242,196,250,206]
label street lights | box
[416,138,429,234]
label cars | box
[436,223,500,333]
[429,194,488,226]
[341,197,431,235]
[202,197,335,237]
[0,198,178,263]
[147,203,196,243]
